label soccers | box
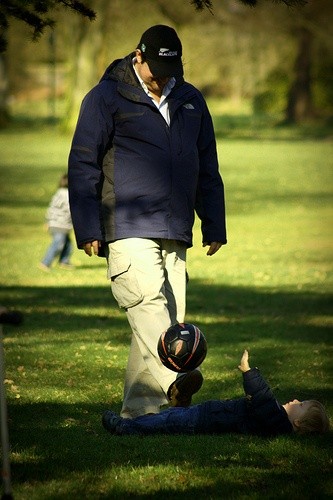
[157,323,209,373]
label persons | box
[67,25,227,419]
[102,350,330,441]
[39,174,74,271]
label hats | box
[135,25,184,80]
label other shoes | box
[101,407,128,438]
[56,264,74,271]
[37,261,50,274]
[166,370,203,409]
